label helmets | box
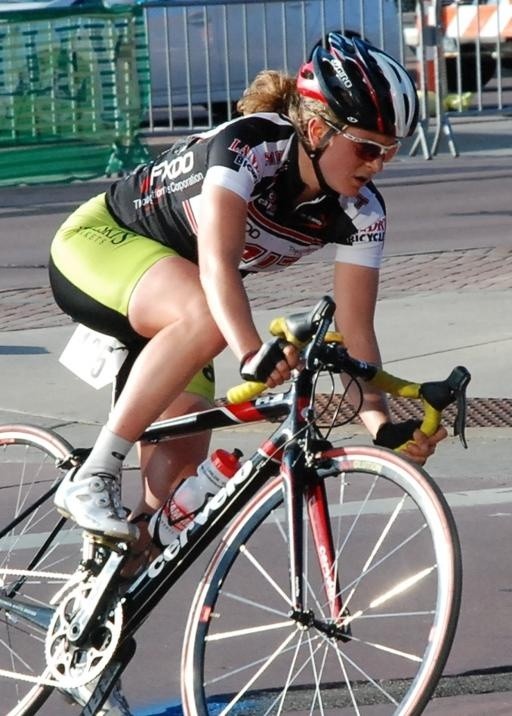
[295,32,419,139]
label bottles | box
[146,449,249,549]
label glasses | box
[326,121,401,162]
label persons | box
[43,28,449,716]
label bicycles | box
[0,295,467,716]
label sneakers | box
[53,465,141,543]
[56,653,133,716]
